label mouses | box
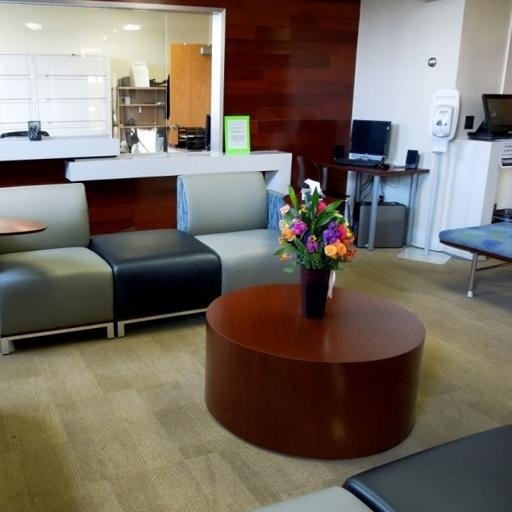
[381,164,390,171]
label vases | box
[298,258,330,320]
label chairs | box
[177,170,301,297]
[1,183,116,355]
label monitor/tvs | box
[467,94,512,141]
[350,120,390,161]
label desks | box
[439,223,510,299]
[309,152,430,253]
[1,216,47,236]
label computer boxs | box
[353,202,407,249]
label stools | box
[344,423,512,512]
[89,227,221,338]
[248,486,372,512]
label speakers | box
[406,150,418,169]
[333,145,344,159]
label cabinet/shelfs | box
[115,86,167,140]
[35,54,114,137]
[2,51,36,132]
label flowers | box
[274,179,356,271]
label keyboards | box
[334,159,380,166]
